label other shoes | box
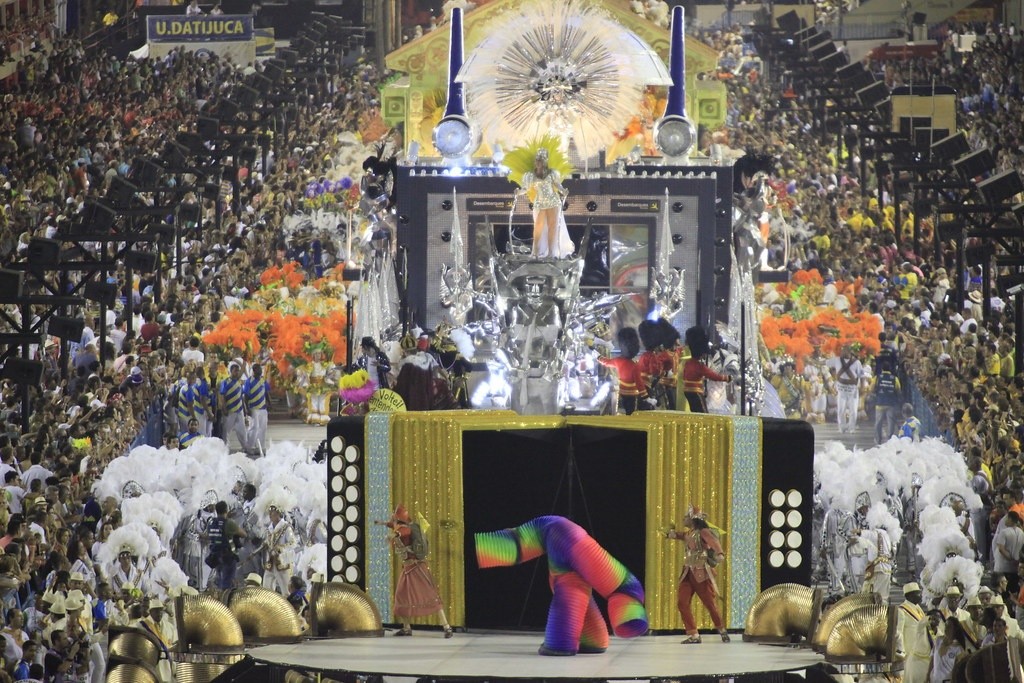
[445,629,453,638]
[393,627,412,636]
[719,631,730,642]
[681,636,701,644]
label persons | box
[514,150,575,260]
[666,505,730,645]
[359,317,464,409]
[0,0,403,682]
[683,19,1023,683]
[592,317,732,415]
[376,503,453,639]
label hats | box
[436,316,452,337]
[96,143,105,147]
[121,582,133,589]
[394,505,409,523]
[71,573,83,581]
[244,572,261,587]
[968,290,982,304]
[43,590,85,613]
[964,346,976,357]
[361,336,374,345]
[937,268,945,276]
[987,595,1005,605]
[146,600,166,613]
[976,586,993,597]
[35,497,49,505]
[967,596,984,606]
[67,197,77,203]
[903,582,921,595]
[688,503,703,520]
[181,585,199,598]
[944,586,962,597]
[399,327,417,351]
